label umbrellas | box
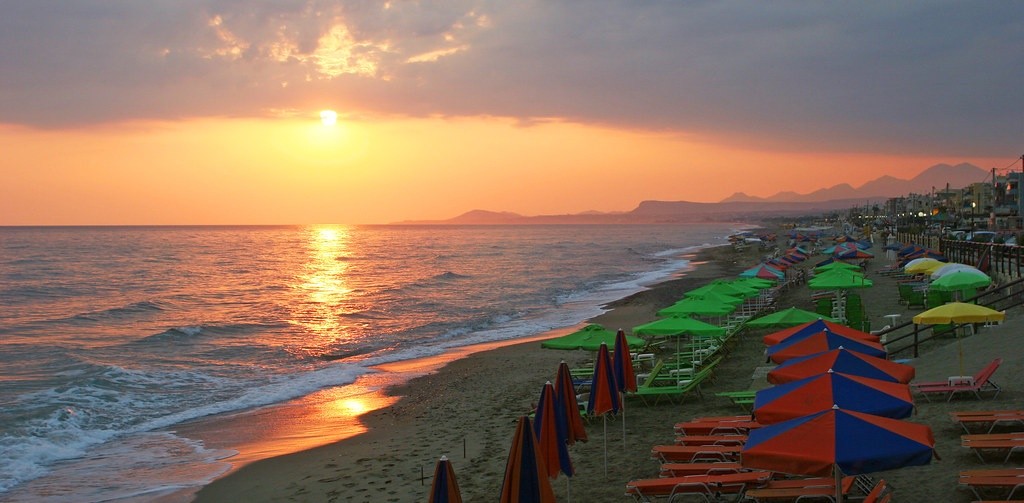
[726,231,876,265]
[587,340,625,480]
[632,314,728,390]
[541,323,647,375]
[741,404,936,503]
[808,261,873,323]
[766,346,916,385]
[761,317,882,345]
[767,328,887,364]
[912,299,1008,400]
[553,360,588,503]
[612,328,639,453]
[658,246,814,371]
[429,454,463,503]
[745,305,847,329]
[885,239,950,263]
[926,211,956,226]
[753,368,914,425]
[532,380,576,478]
[901,257,991,301]
[498,415,558,503]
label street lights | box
[970,202,977,242]
[919,212,924,238]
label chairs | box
[527,260,1024,503]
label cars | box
[927,223,1023,255]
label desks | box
[704,339,715,342]
[691,359,701,364]
[637,373,651,386]
[947,375,974,401]
[629,353,638,360]
[737,315,749,319]
[720,325,735,330]
[678,380,692,386]
[694,348,708,355]
[669,368,693,379]
[637,354,655,368]
